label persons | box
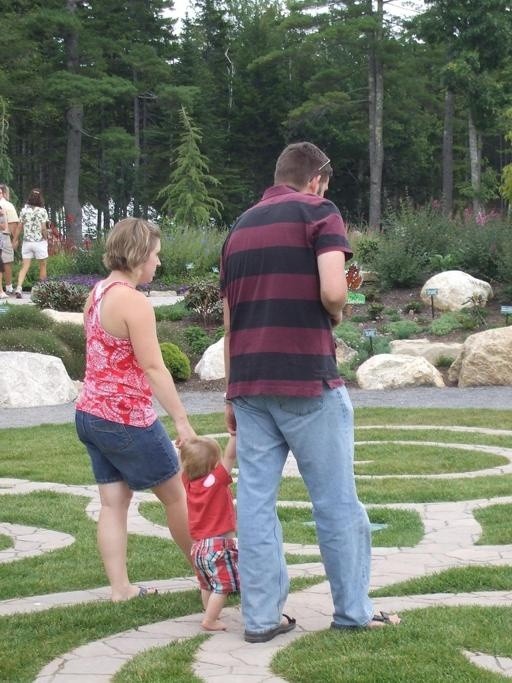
[73,217,198,601]
[177,431,241,632]
[0,183,49,300]
[221,141,402,643]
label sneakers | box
[0,284,22,299]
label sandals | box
[138,582,158,598]
[330,611,399,631]
[244,613,296,643]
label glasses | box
[319,157,330,171]
